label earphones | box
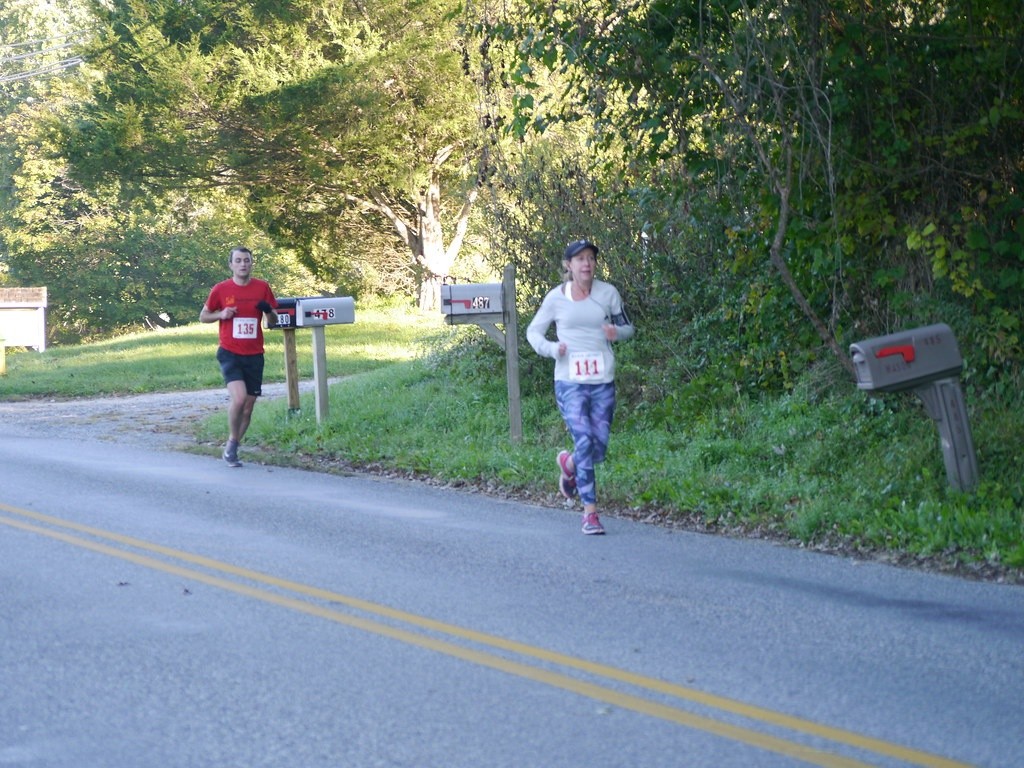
[568,264,571,268]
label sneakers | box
[582,512,605,535]
[223,438,243,467]
[557,449,577,498]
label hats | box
[566,241,599,260]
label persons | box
[526,241,634,534]
[199,248,278,467]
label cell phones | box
[621,301,631,325]
